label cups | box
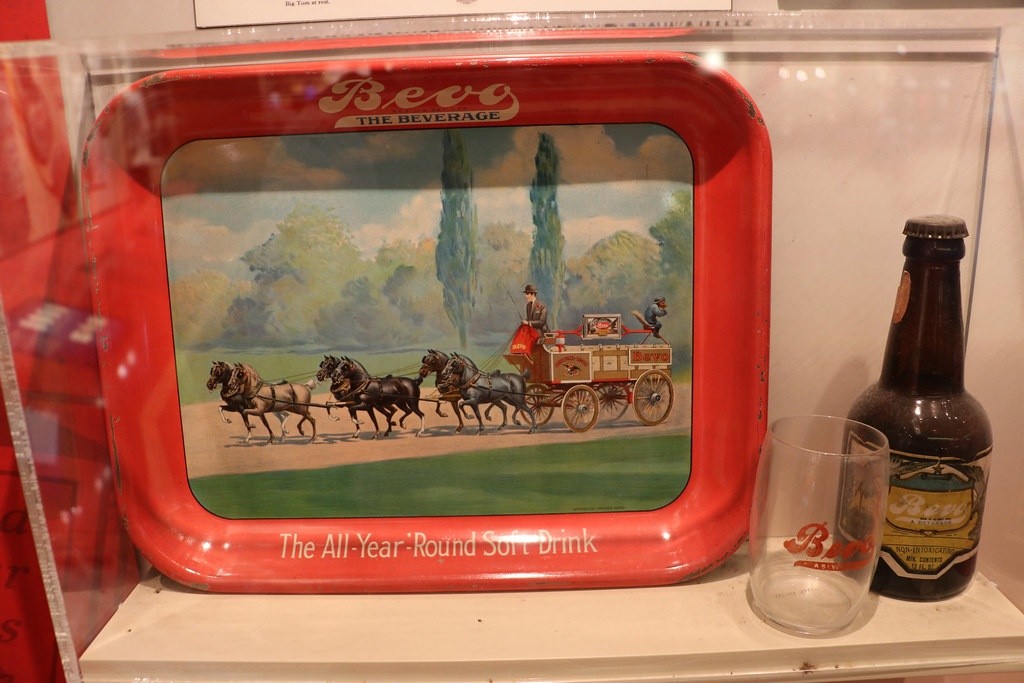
[748,416,887,639]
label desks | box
[77,564,1023,683]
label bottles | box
[834,217,992,602]
